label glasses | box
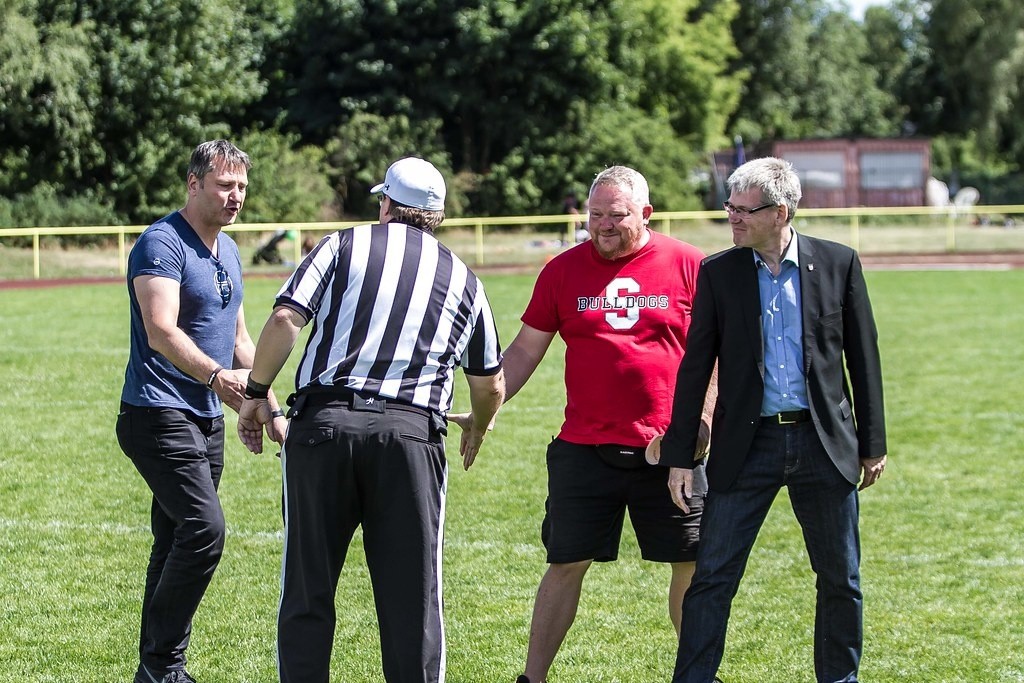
[217,261,233,306]
[724,201,774,218]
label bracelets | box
[243,370,271,400]
[206,366,224,391]
[272,410,286,419]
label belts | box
[758,409,812,424]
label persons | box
[668,156,888,683]
[502,164,720,683]
[237,156,508,683]
[116,139,287,683]
[561,191,589,234]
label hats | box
[370,156,446,211]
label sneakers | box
[132,662,196,683]
